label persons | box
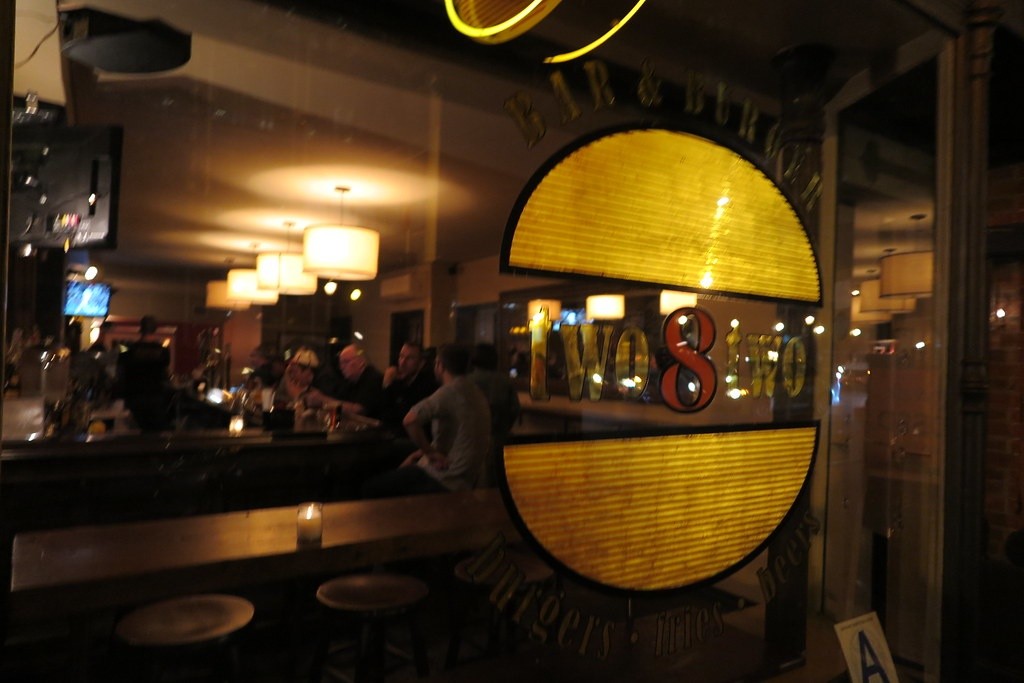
[312,342,380,415]
[112,315,174,407]
[387,342,492,496]
[290,350,345,410]
[306,339,442,471]
[89,321,124,360]
[465,342,521,442]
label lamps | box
[845,288,887,328]
[204,267,251,311]
[859,256,924,318]
[881,211,940,305]
[524,273,563,321]
[225,245,278,308]
[303,184,382,283]
[256,222,319,297]
[653,284,699,314]
[584,277,629,324]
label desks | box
[10,490,514,611]
[2,383,378,519]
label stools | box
[314,575,430,682]
[119,593,257,683]
